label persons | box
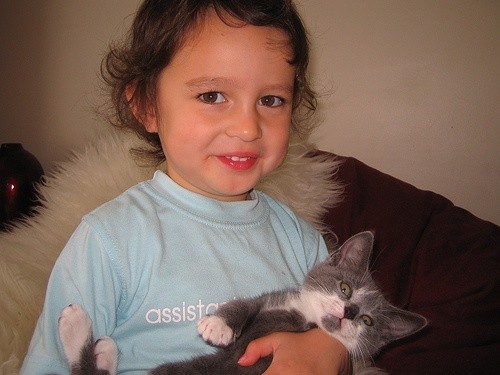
[19,0,356,375]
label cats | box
[55,228,431,375]
